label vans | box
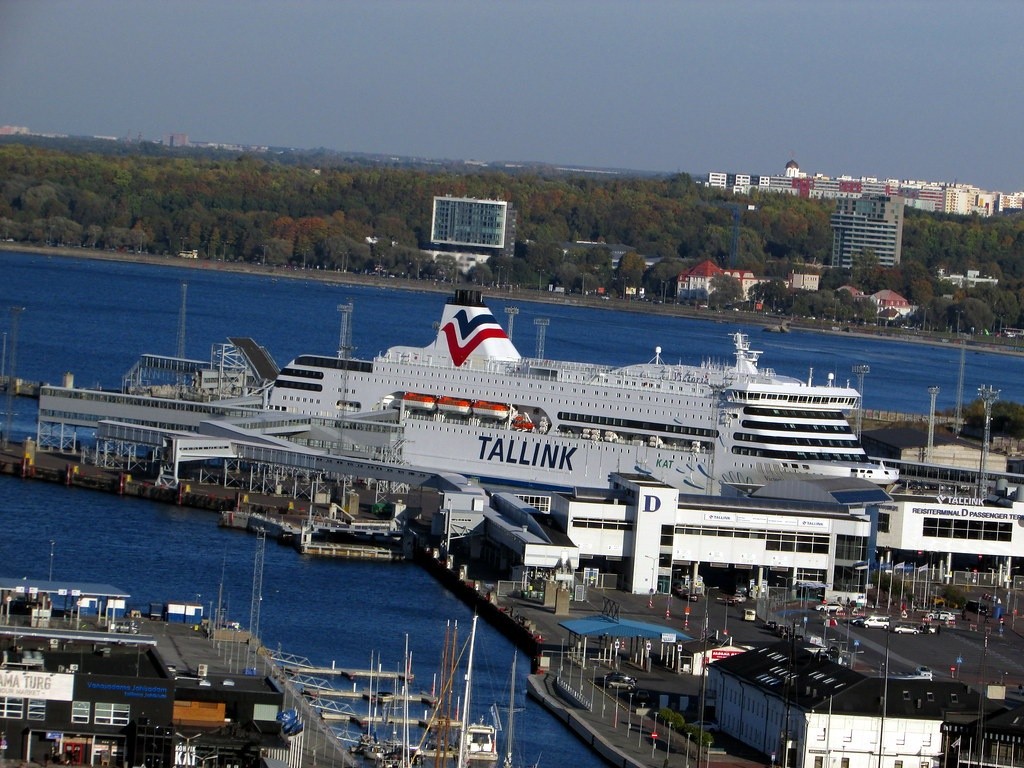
[114,619,139,633]
[967,600,988,615]
[861,615,889,630]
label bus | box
[741,607,756,622]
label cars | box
[722,584,748,607]
[915,665,933,679]
[917,625,936,634]
[602,671,636,690]
[925,611,956,621]
[892,624,920,634]
[850,616,866,626]
[815,602,843,612]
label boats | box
[466,714,499,762]
[438,395,471,415]
[403,392,437,411]
[468,401,508,419]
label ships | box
[199,286,904,497]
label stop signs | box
[951,666,956,671]
[918,551,923,555]
[651,732,658,738]
[979,555,983,560]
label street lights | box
[684,732,693,768]
[176,732,202,768]
[559,636,647,747]
[706,740,713,768]
[191,753,220,768]
[705,586,720,621]
[652,712,659,758]
[988,566,1004,606]
[664,721,673,768]
[644,555,665,607]
[659,567,682,620]
[818,593,828,642]
